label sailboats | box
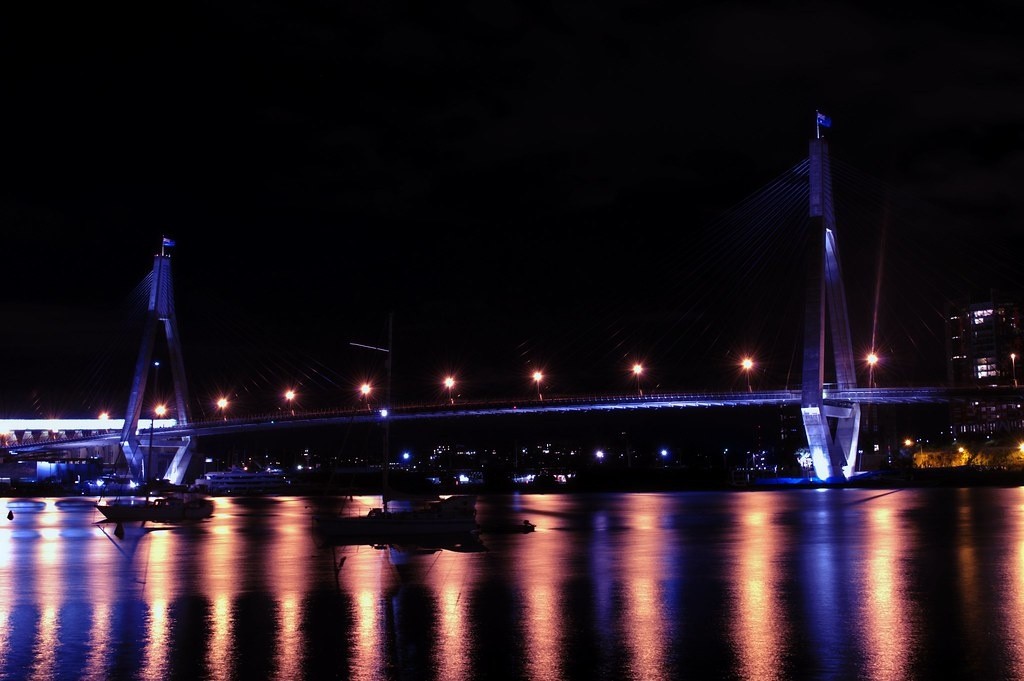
[312,310,480,540]
[91,360,215,523]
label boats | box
[474,520,535,535]
[193,455,296,497]
